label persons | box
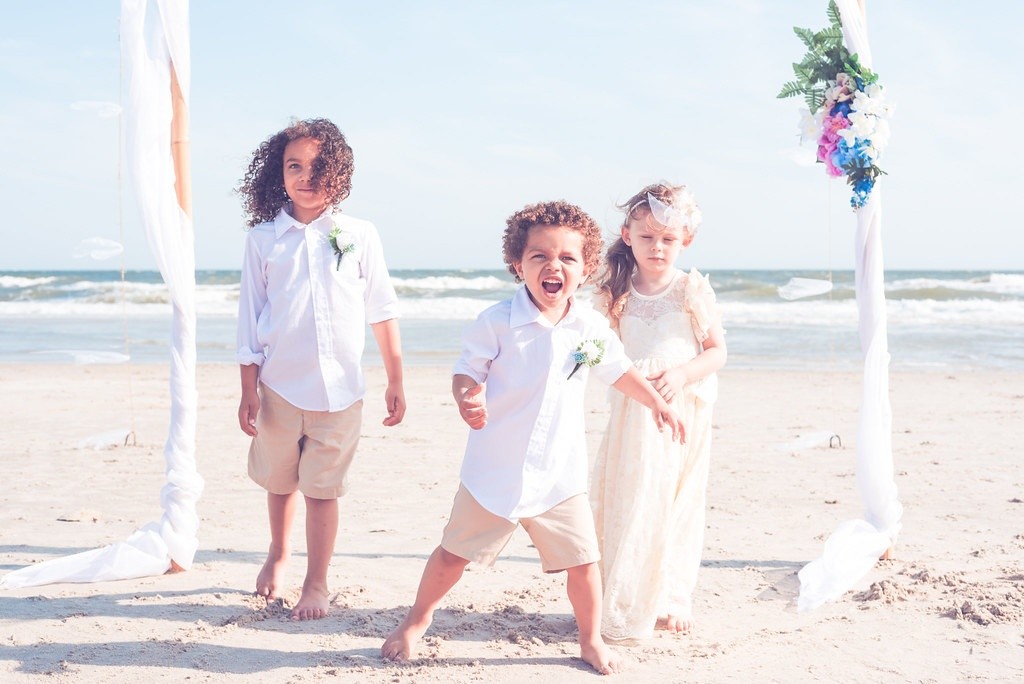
[233,116,407,621]
[382,201,688,674]
[587,183,729,641]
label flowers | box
[776,0,893,208]
[327,225,358,271]
[567,337,606,382]
